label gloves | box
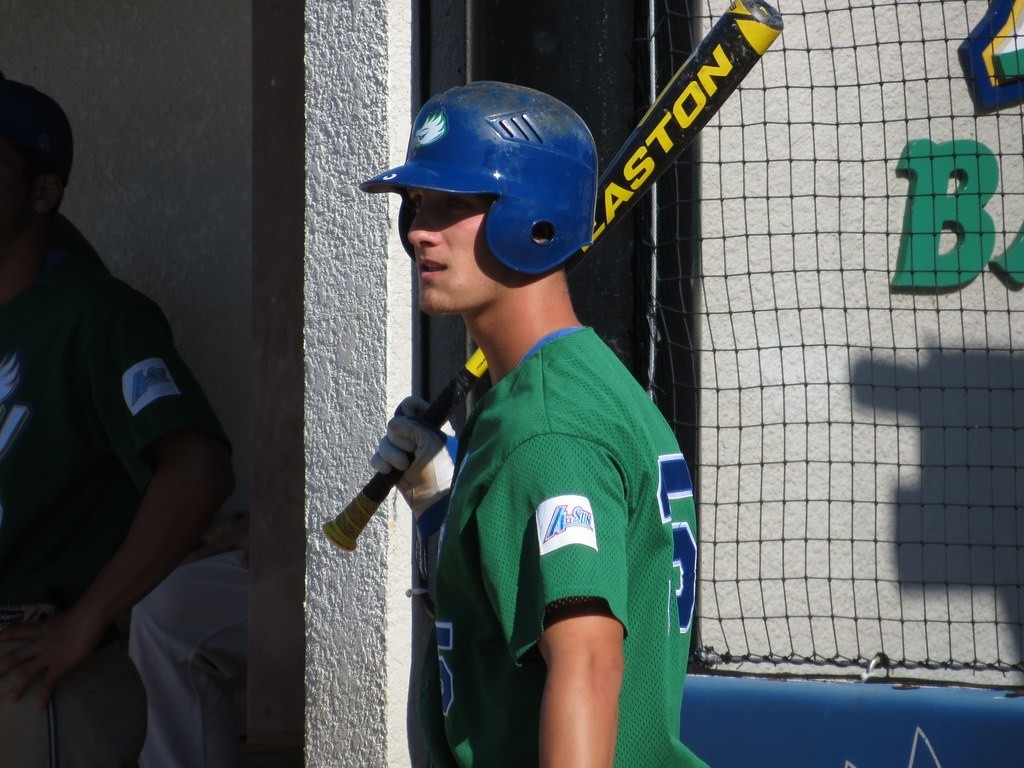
[369,397,459,548]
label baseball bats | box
[319,0,785,553]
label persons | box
[1,73,269,768]
[357,80,701,768]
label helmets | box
[358,80,598,276]
